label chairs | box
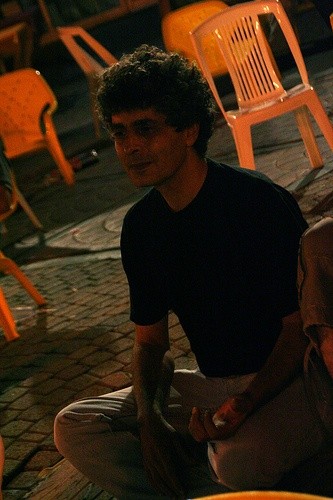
[0,67,73,343]
[162,0,333,171]
[58,26,120,139]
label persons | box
[298,217,333,446]
[0,137,13,214]
[52,44,322,496]
